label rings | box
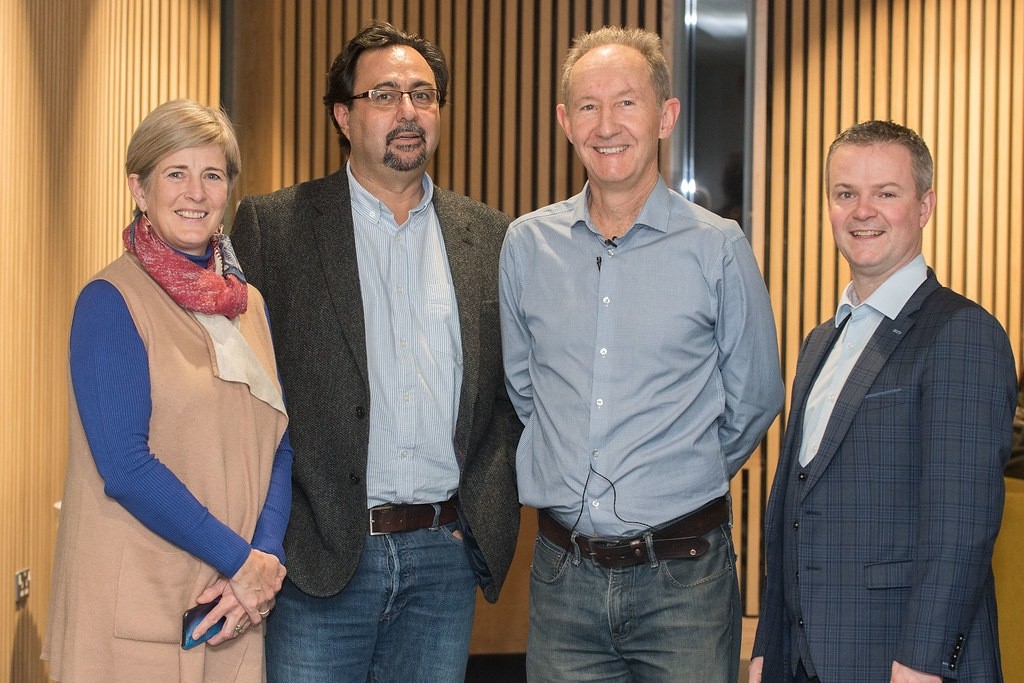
[259,607,270,615]
[235,624,242,634]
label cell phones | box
[182,595,227,650]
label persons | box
[39,98,296,683]
[228,19,522,683]
[749,120,1016,683]
[499,24,783,683]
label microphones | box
[606,236,619,249]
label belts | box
[368,503,458,536]
[537,496,729,570]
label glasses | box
[346,88,442,108]
